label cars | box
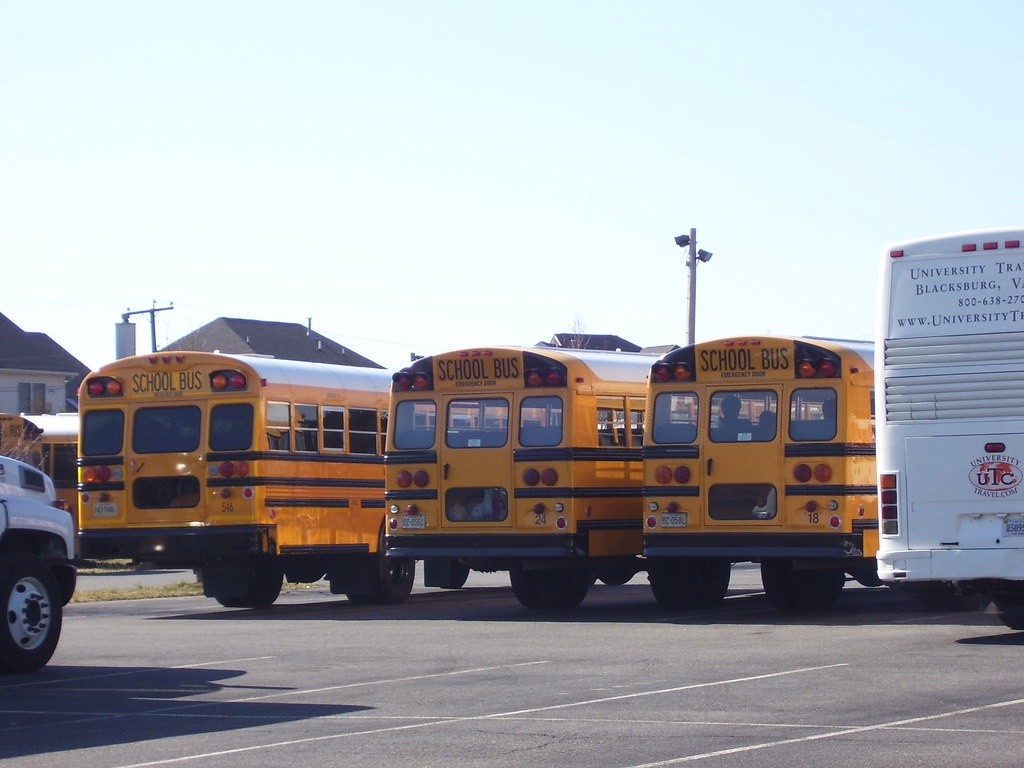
[0,454,78,675]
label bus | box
[383,346,824,610]
[0,413,80,563]
[75,349,565,607]
[870,226,1024,601]
[641,333,875,612]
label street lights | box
[674,228,714,346]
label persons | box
[716,396,836,428]
